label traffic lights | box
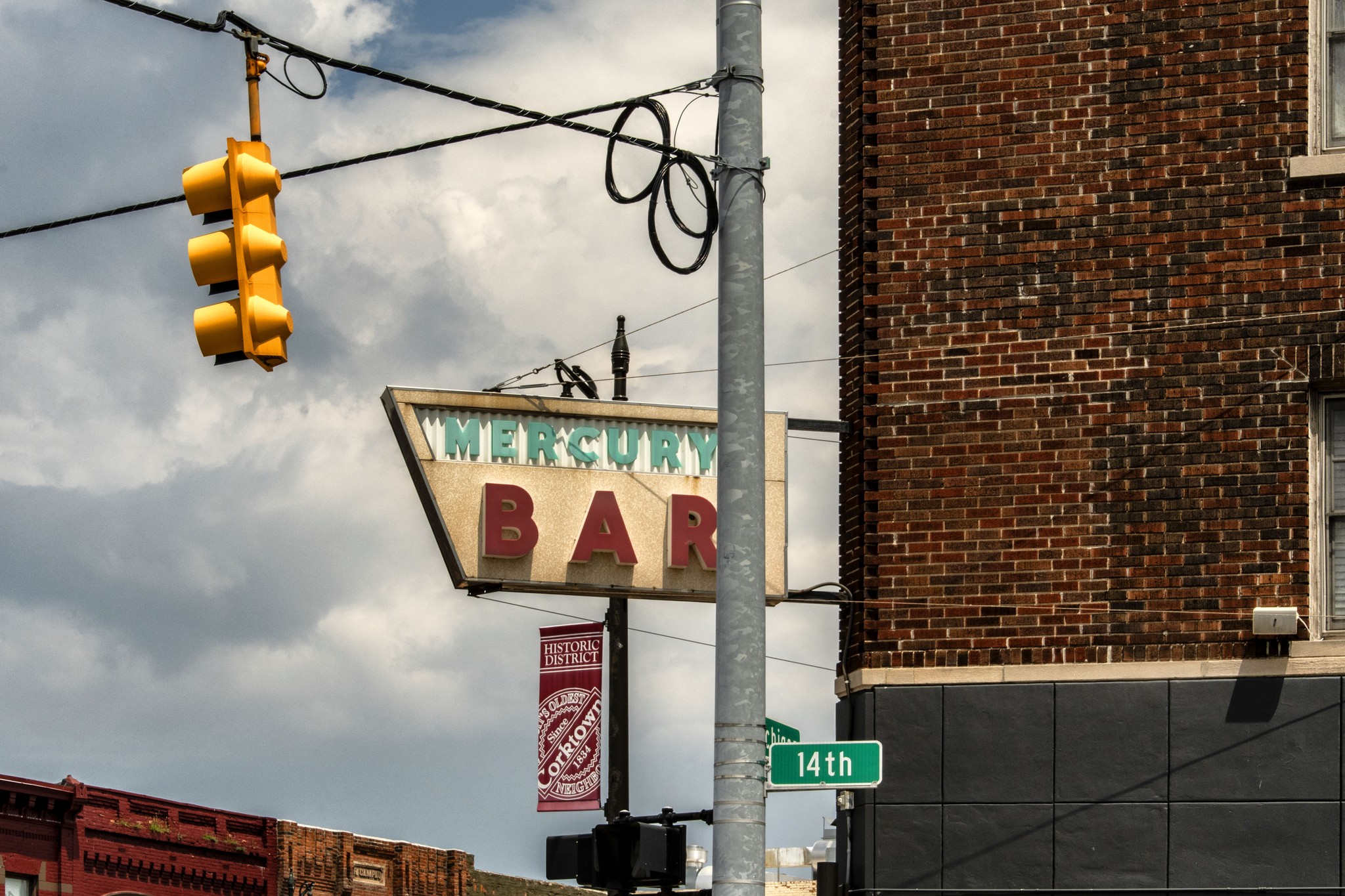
[181,139,294,373]
[592,823,683,889]
